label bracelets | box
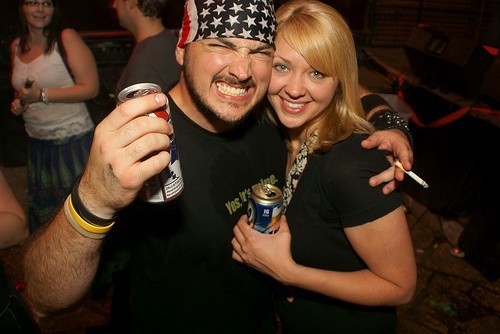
[40,87,49,104]
[368,109,393,122]
[371,112,410,133]
[68,196,116,233]
[64,200,107,239]
[360,93,394,115]
[71,176,119,226]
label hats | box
[177,0,278,51]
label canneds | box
[117,82,184,203]
[247,183,283,235]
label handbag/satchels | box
[56,24,117,127]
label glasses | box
[22,1,55,9]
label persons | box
[22,0,414,334]
[112,0,185,108]
[0,170,43,334]
[9,0,101,236]
[232,0,417,334]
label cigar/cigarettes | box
[394,161,429,189]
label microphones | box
[21,76,35,106]
